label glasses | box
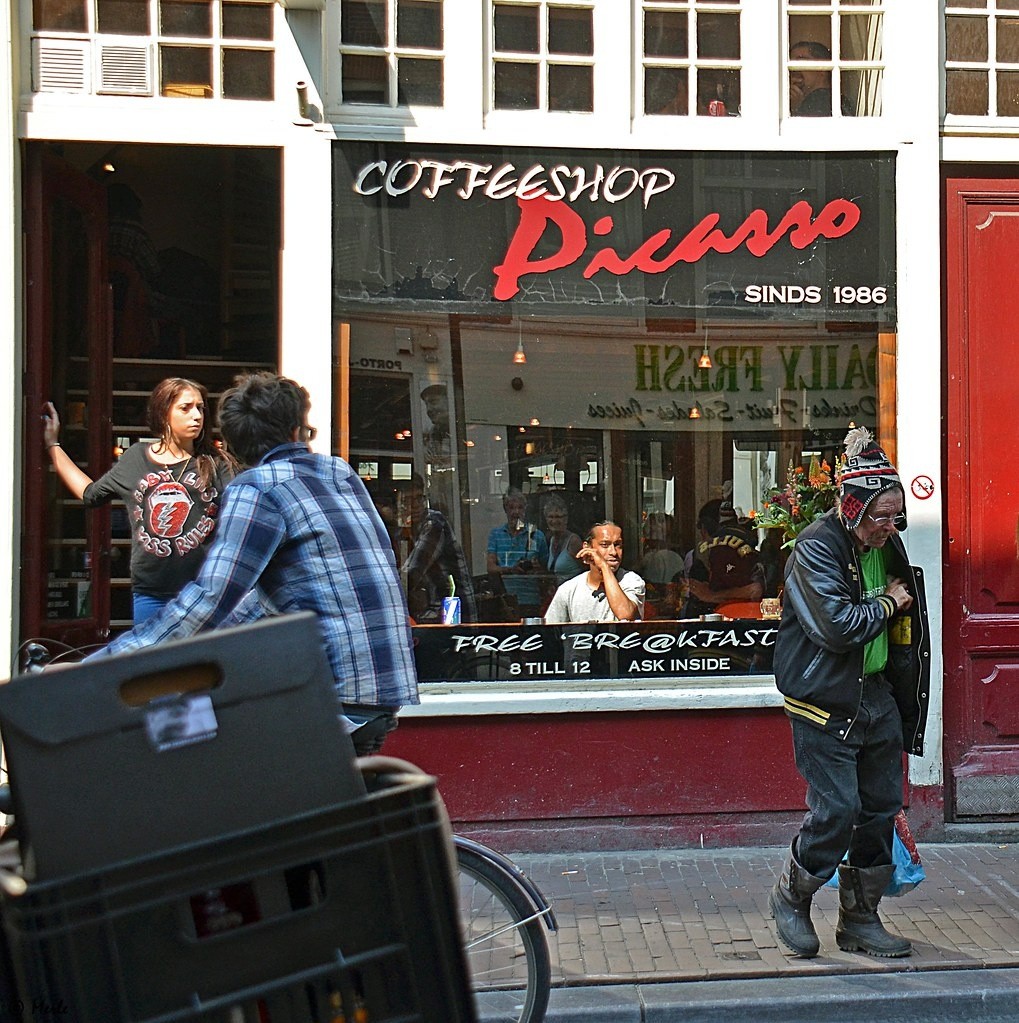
[545,514,566,521]
[298,423,317,442]
[398,493,425,504]
[863,511,905,526]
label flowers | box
[748,451,847,549]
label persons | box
[420,384,451,464]
[487,488,581,622]
[392,471,476,624]
[768,425,931,957]
[789,41,854,117]
[42,370,421,758]
[683,499,762,619]
[544,519,645,623]
[45,378,238,625]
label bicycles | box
[1,636,559,1023]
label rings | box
[692,586,695,589]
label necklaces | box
[162,457,190,485]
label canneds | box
[442,596,461,625]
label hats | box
[839,427,909,531]
[697,499,757,537]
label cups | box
[760,598,781,620]
[705,614,724,621]
[523,616,547,624]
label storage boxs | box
[0,773,481,1023]
[0,607,369,884]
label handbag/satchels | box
[822,810,927,897]
[538,573,557,605]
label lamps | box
[363,462,373,481]
[512,320,527,365]
[543,464,550,482]
[689,384,702,419]
[697,318,713,369]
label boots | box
[768,836,830,956]
[836,860,912,957]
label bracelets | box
[46,443,60,449]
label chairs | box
[710,599,783,619]
